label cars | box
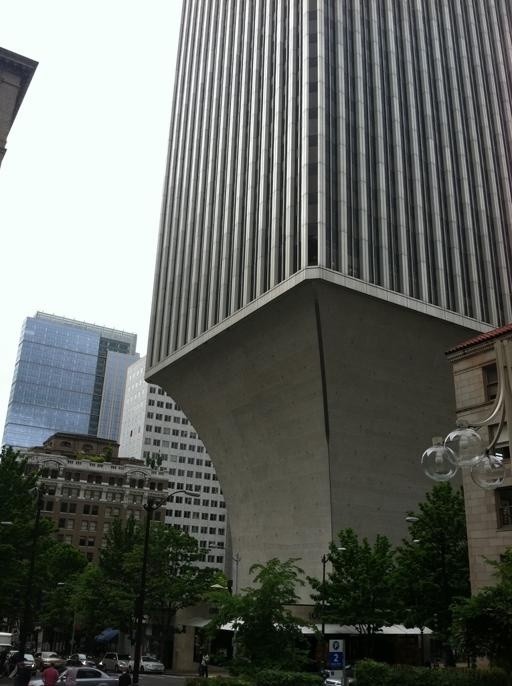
[0,632,165,686]
[320,665,354,686]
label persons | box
[119,666,132,684]
[65,664,79,685]
[201,651,209,678]
[8,657,32,686]
[41,662,59,686]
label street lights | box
[420,339,512,490]
[405,517,453,599]
[320,547,347,656]
[208,544,242,660]
[132,489,201,686]
[20,483,50,649]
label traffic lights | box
[226,579,233,593]
